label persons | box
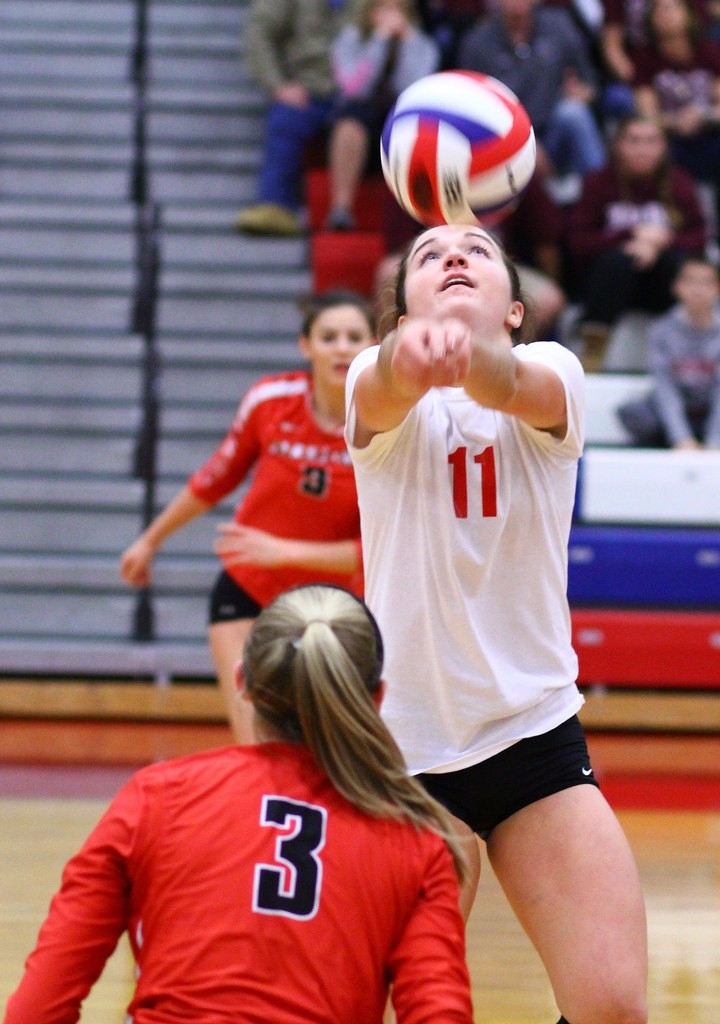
[342,224,651,1024]
[237,0,720,453]
[2,584,478,1024]
[124,290,376,746]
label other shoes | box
[333,204,351,230]
[237,196,300,232]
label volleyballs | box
[381,69,536,229]
